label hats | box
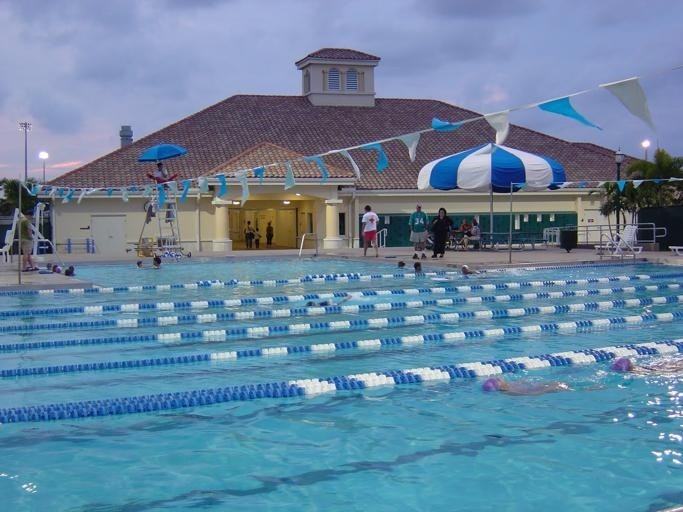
[417,203,421,207]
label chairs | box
[0,230,13,264]
[595,225,643,255]
[669,246,683,255]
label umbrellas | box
[137,143,187,165]
[418,140,567,247]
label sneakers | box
[412,253,419,258]
[432,255,437,258]
[422,253,426,258]
[440,255,443,257]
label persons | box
[152,258,162,268]
[361,205,379,257]
[45,263,75,277]
[397,260,406,268]
[14,209,40,271]
[460,265,474,274]
[254,227,261,248]
[136,258,145,268]
[152,163,169,190]
[409,202,430,262]
[458,219,481,251]
[243,219,255,248]
[413,262,422,271]
[265,221,273,248]
[430,207,453,257]
[460,219,471,232]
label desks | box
[449,232,521,238]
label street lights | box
[17,121,33,180]
[641,139,651,160]
[614,144,625,243]
[38,151,49,186]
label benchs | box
[425,238,548,250]
